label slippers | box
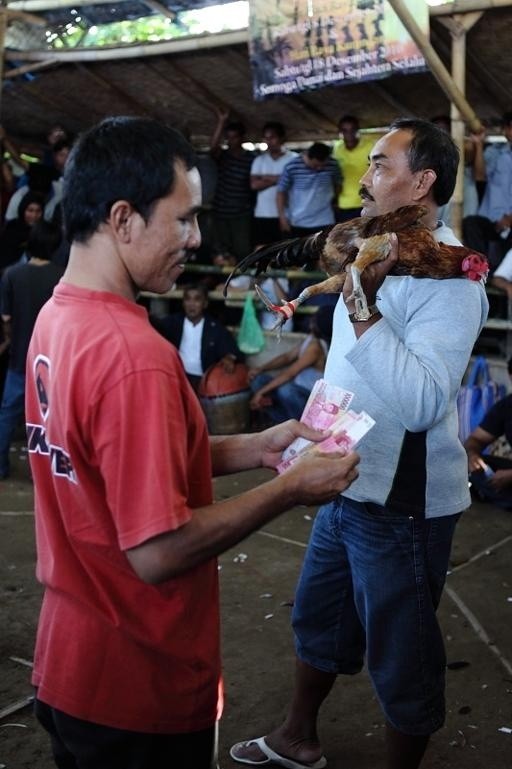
[230,734,329,767]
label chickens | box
[222,204,490,343]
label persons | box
[230,120,489,766]
[0,116,511,509]
[31,118,360,769]
